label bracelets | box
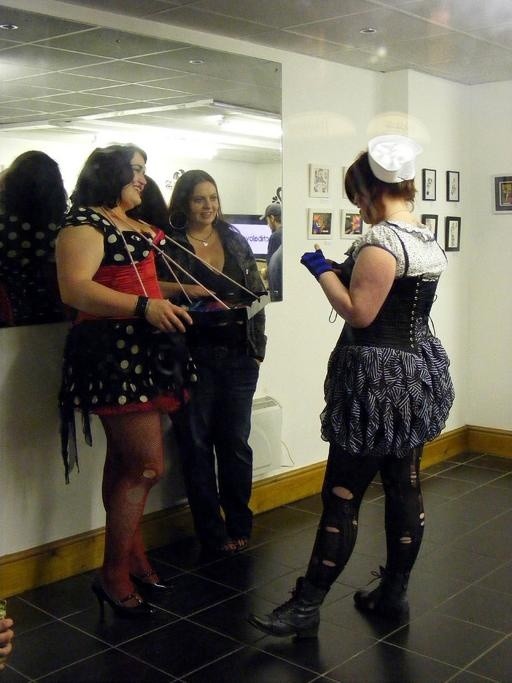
[135,295,148,317]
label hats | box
[367,135,423,185]
[258,202,281,220]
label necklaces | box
[188,229,216,246]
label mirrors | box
[1,4,283,328]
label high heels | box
[95,571,150,619]
[130,570,172,593]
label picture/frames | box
[445,215,461,251]
[341,208,367,240]
[493,173,512,214]
[342,166,350,199]
[446,170,459,202]
[421,214,438,242]
[309,163,331,198]
[307,208,335,240]
[421,168,436,201]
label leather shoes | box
[353,565,411,625]
[248,575,331,639]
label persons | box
[0,150,68,328]
[125,175,172,235]
[247,134,448,644]
[260,204,282,301]
[154,169,267,554]
[55,144,218,624]
[0,618,15,672]
